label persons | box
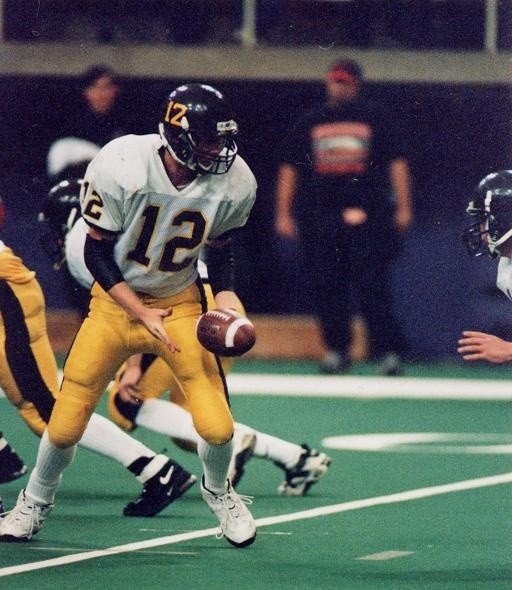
[0,239,196,516]
[0,83,256,547]
[273,59,412,373]
[456,169,512,365]
[37,180,334,496]
[45,66,144,187]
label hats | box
[322,60,362,83]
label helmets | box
[459,169,512,260]
[42,179,84,237]
[157,83,238,177]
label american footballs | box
[197,308,257,357]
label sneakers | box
[200,474,257,548]
[278,445,330,496]
[123,459,196,516]
[382,352,402,374]
[0,442,54,542]
[227,434,257,486]
[321,352,349,373]
